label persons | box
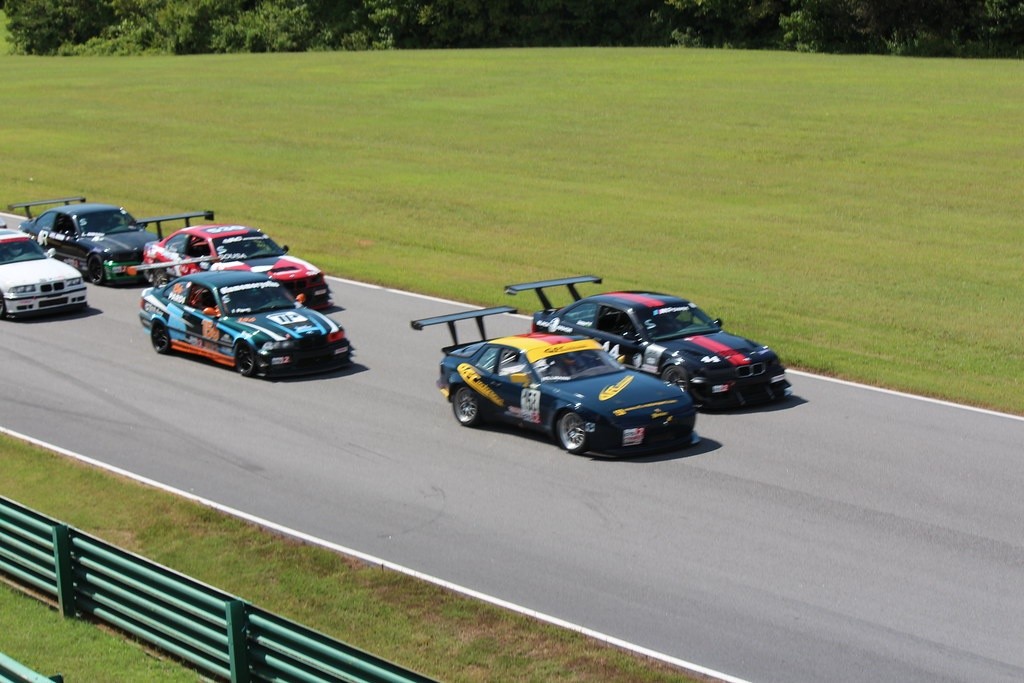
[550,353,586,377]
[0,242,26,261]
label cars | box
[407,303,704,460]
[507,273,794,413]
[0,223,87,321]
[133,210,334,312]
[7,194,165,288]
[124,254,355,380]
